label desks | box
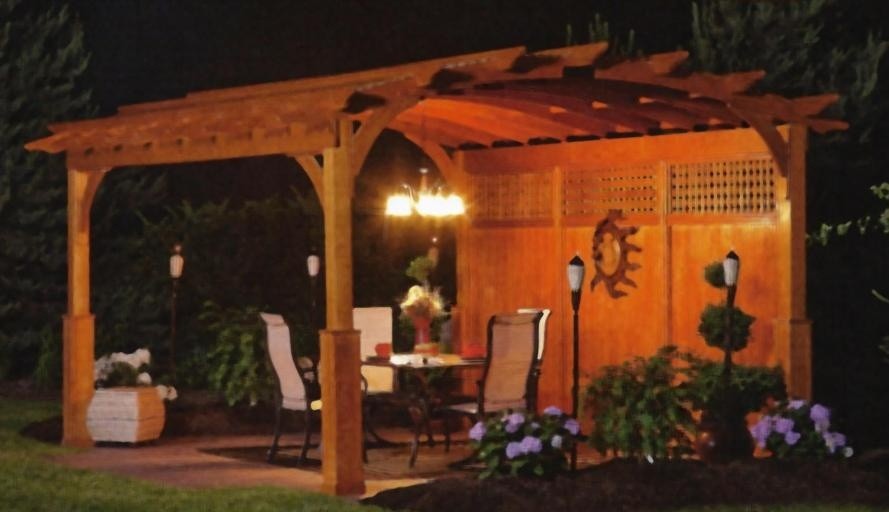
[356,350,485,467]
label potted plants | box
[398,285,449,354]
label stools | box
[84,385,167,446]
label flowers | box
[466,403,583,484]
[748,395,849,472]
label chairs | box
[258,302,398,464]
[436,307,556,458]
